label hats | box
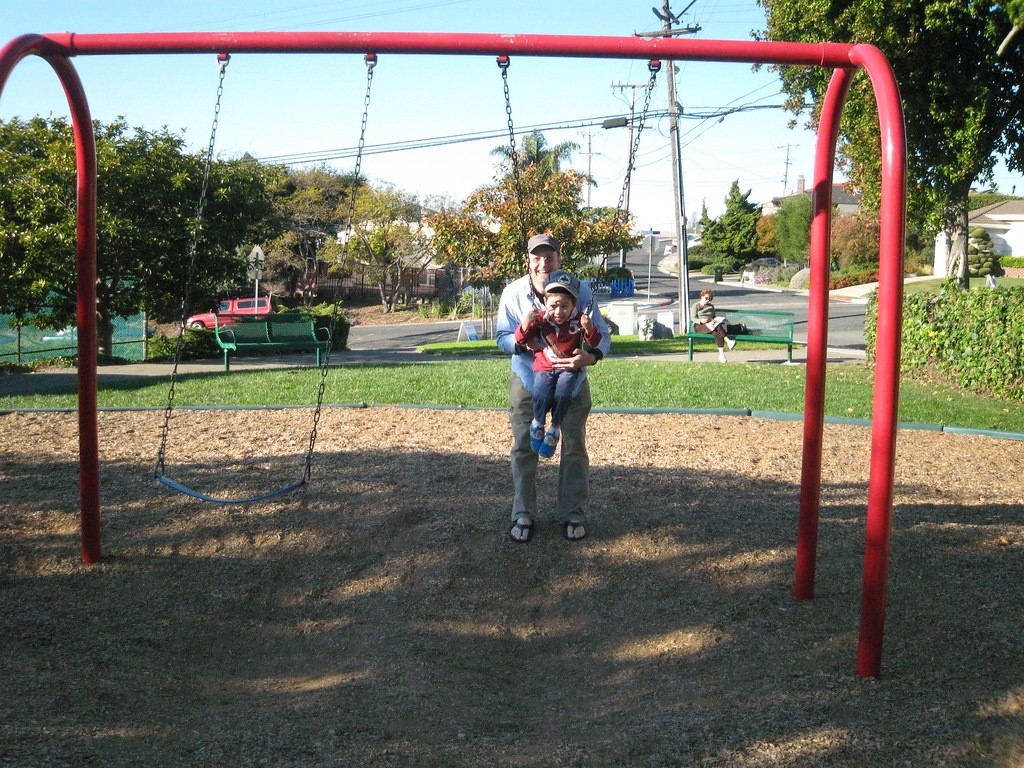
[542,269,580,299]
[528,233,559,253]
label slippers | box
[509,519,535,543]
[567,520,586,541]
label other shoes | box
[728,340,736,351]
[719,355,727,363]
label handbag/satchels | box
[726,323,748,335]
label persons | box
[690,289,737,364]
[495,233,612,542]
[514,268,602,457]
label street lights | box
[600,109,691,333]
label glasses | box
[703,296,712,302]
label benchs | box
[685,307,795,363]
[214,313,332,372]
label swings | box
[151,50,379,507]
[494,55,664,405]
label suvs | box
[186,297,277,331]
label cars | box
[744,257,782,272]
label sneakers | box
[540,431,559,458]
[530,424,545,453]
